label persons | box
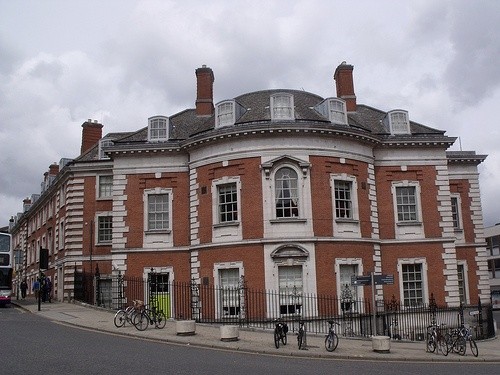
[32,276,54,303]
[20,278,28,301]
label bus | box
[0,232,14,307]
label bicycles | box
[33,284,52,304]
[296,321,309,350]
[324,320,341,352]
[271,318,288,349]
[426,323,483,357]
[133,303,167,331]
[113,299,144,328]
